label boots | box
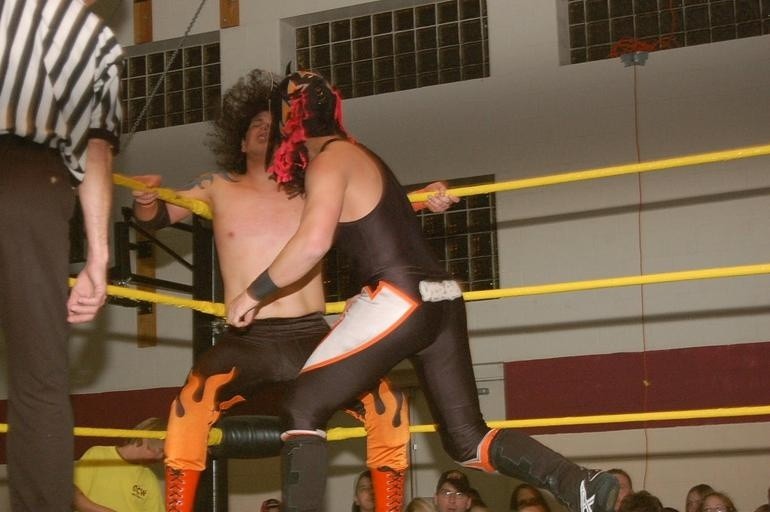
[163,464,202,512]
[277,428,330,512]
[369,466,407,512]
[486,425,621,512]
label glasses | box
[439,490,465,498]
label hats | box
[437,469,470,492]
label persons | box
[0,0,125,512]
[73,417,168,512]
[351,470,375,512]
[132,73,461,512]
[260,499,282,512]
[223,72,620,512]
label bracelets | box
[246,269,280,302]
